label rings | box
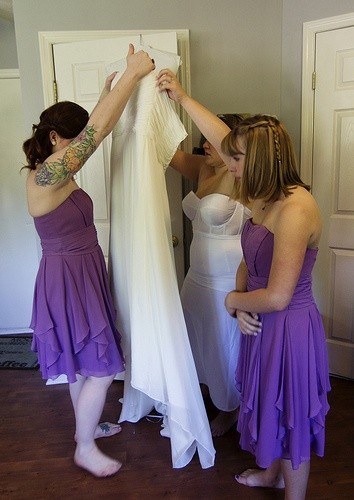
[151,59,154,64]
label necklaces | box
[261,202,269,211]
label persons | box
[219,114,331,500]
[155,66,249,439]
[18,42,156,479]
